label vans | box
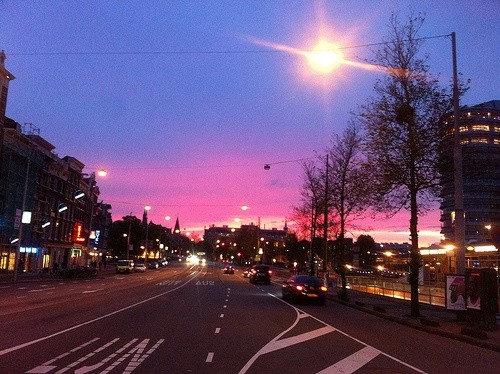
[117,259,133,274]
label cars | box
[186,254,207,263]
[243,265,273,285]
[281,274,327,306]
[148,259,169,270]
[135,264,147,273]
[223,265,234,274]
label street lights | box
[127,205,151,259]
[306,30,467,320]
[13,159,107,282]
[241,204,261,264]
[263,154,328,274]
